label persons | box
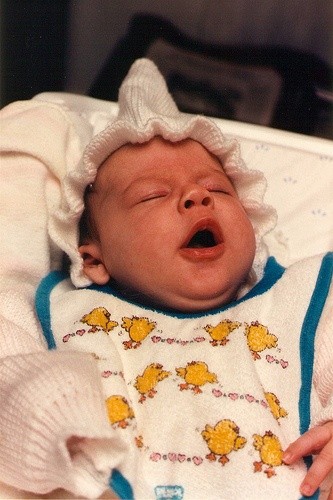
[0,58,333,500]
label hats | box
[46,56,281,292]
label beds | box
[0,93,333,500]
